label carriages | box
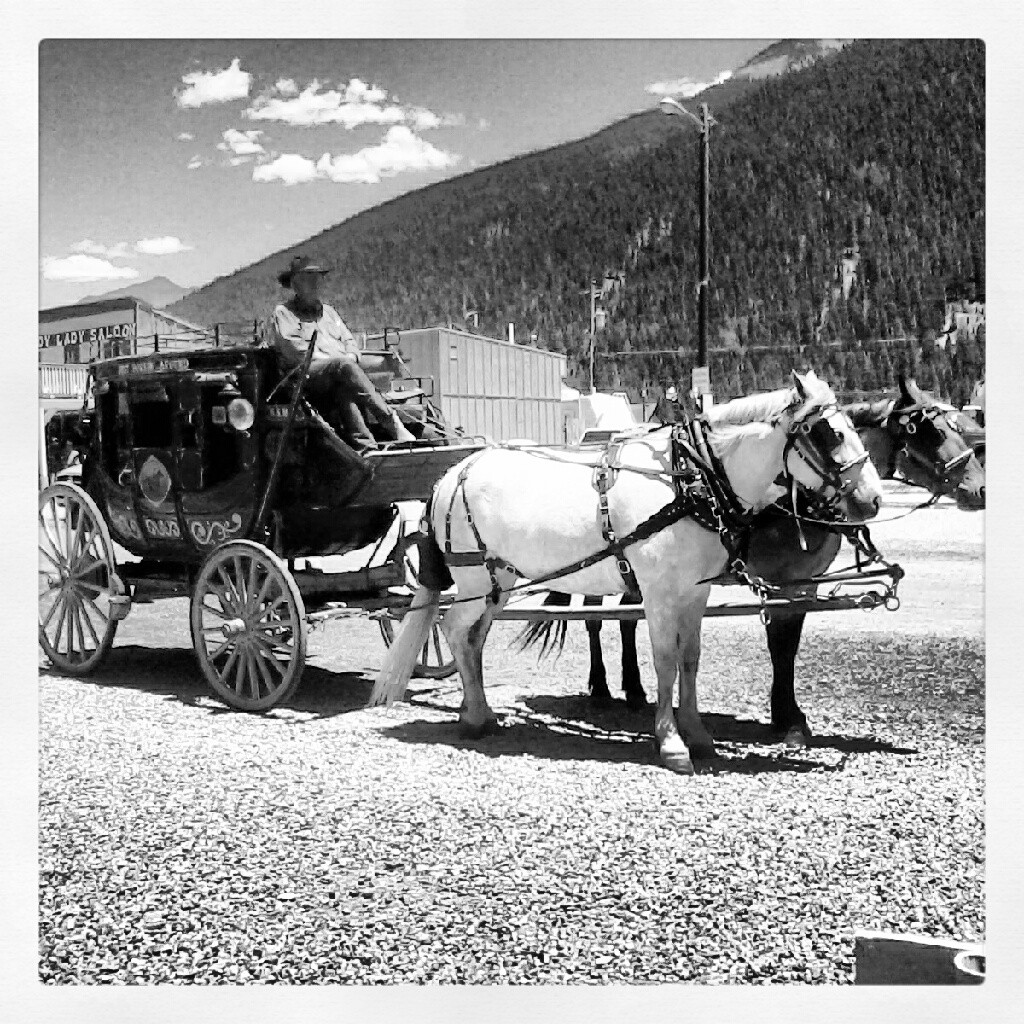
[37,339,986,775]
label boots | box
[382,410,416,441]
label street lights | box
[660,97,708,366]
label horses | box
[505,353,985,749]
[365,370,885,776]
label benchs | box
[255,346,434,425]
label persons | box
[262,254,416,448]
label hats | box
[278,256,329,288]
